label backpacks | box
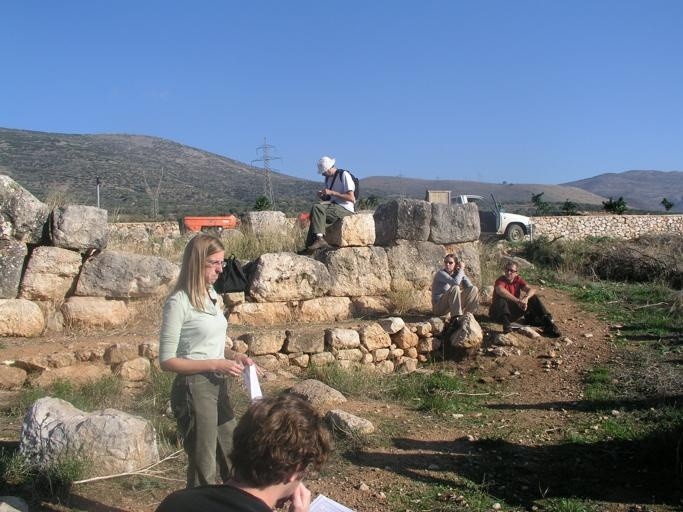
[326,169,359,200]
[216,255,248,292]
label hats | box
[317,157,336,174]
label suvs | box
[451,193,535,242]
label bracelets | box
[524,295,528,297]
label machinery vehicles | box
[177,214,239,235]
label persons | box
[155,392,335,512]
[487,260,561,338]
[297,157,356,255]
[432,254,480,323]
[158,234,264,488]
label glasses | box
[445,261,453,264]
[505,268,517,272]
[207,261,226,268]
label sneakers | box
[503,321,512,333]
[543,324,562,337]
[297,248,318,256]
[308,237,328,250]
